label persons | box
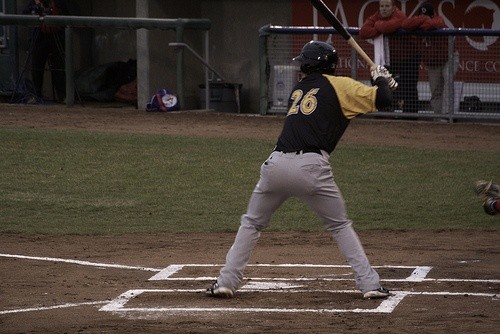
[206,40,390,297]
[402,3,460,122]
[23,0,65,105]
[359,0,408,113]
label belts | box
[275,148,321,155]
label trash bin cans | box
[195,79,245,114]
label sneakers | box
[363,287,390,298]
[206,281,233,298]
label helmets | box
[292,41,337,74]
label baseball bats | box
[309,0,377,75]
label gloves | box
[483,198,499,215]
[371,66,392,81]
